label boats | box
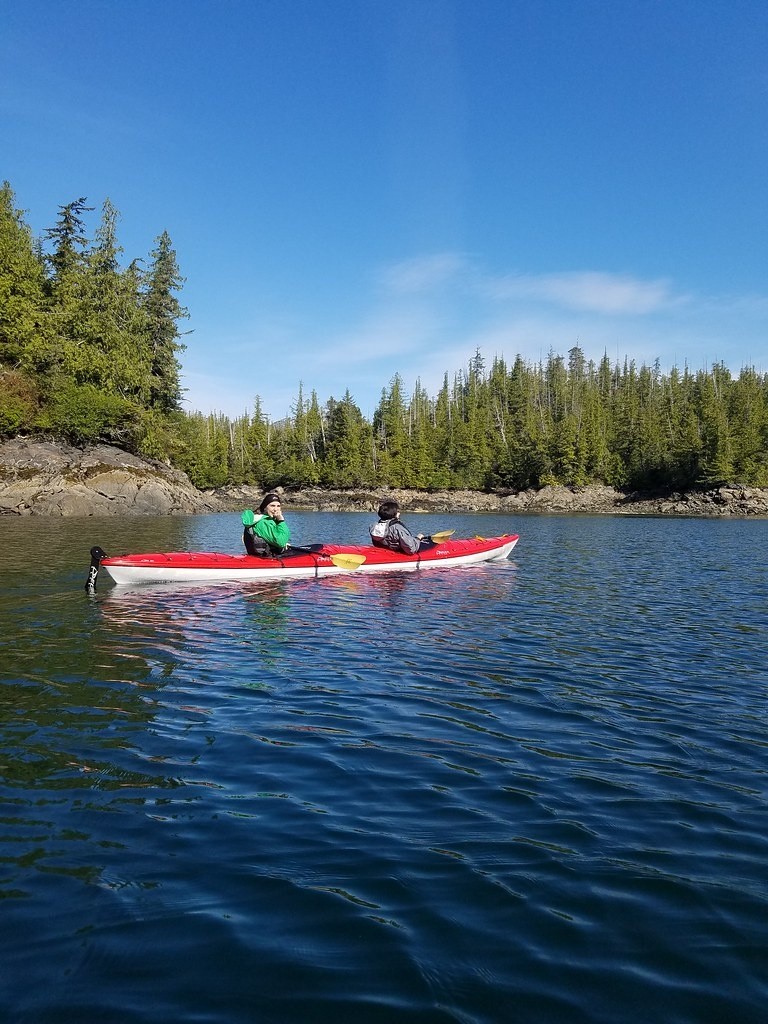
[89,531,519,586]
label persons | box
[241,494,294,557]
[369,502,424,555]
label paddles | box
[421,528,456,543]
[289,546,368,571]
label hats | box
[260,494,280,513]
